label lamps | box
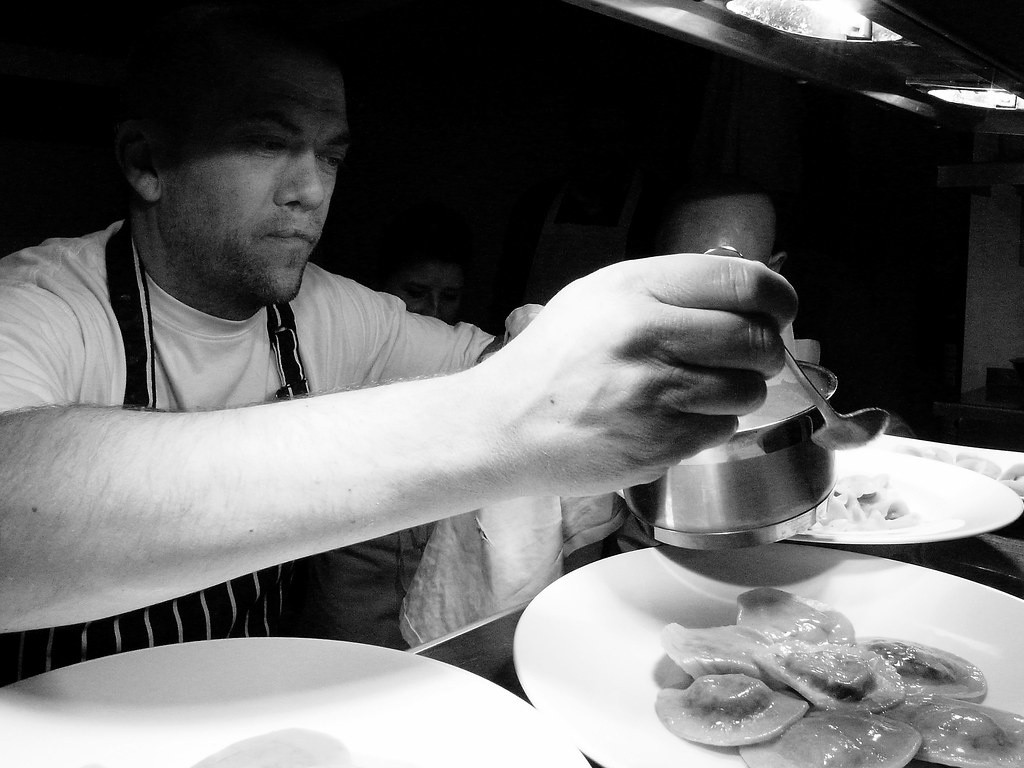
[927,80,1024,111]
[725,0,905,45]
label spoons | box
[703,246,891,452]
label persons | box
[368,205,472,331]
[642,172,787,278]
[0,0,805,693]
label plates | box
[0,638,595,768]
[784,448,1024,545]
[513,541,1024,768]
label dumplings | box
[654,588,1024,767]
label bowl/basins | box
[623,357,838,552]
